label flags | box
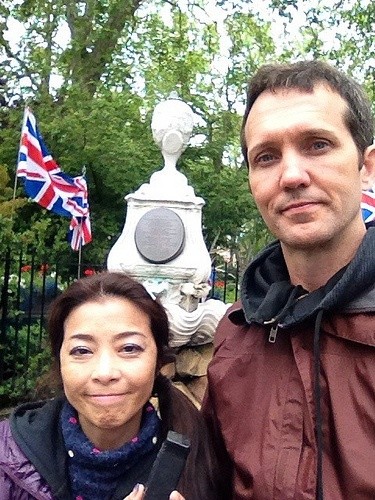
[67,175,92,252]
[359,189,374,223]
[16,108,87,219]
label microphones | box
[141,430,192,500]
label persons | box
[202,62,375,499]
[0,274,211,500]
